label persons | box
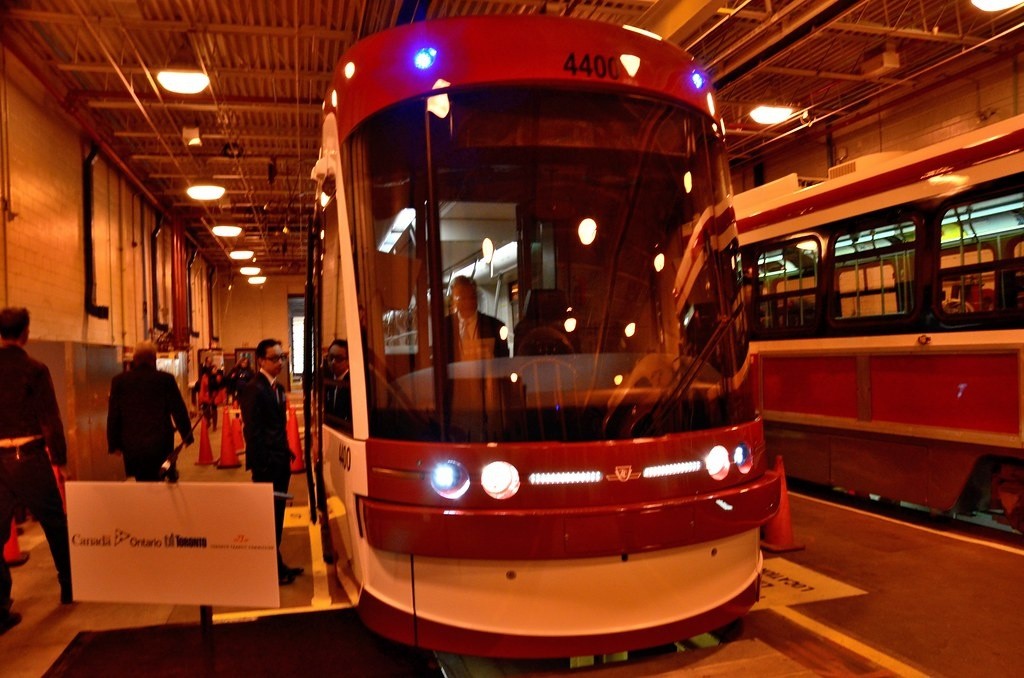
[444,275,510,363]
[226,353,254,400]
[239,340,305,586]
[0,307,73,634]
[200,357,223,431]
[961,275,994,312]
[326,339,352,420]
[106,341,194,481]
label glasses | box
[326,353,346,363]
[452,295,472,302]
[263,352,286,363]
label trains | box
[305,11,782,672]
[733,110,1024,538]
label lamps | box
[157,38,267,284]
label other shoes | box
[6,551,29,566]
[281,561,304,573]
[60,589,72,603]
[16,527,24,535]
[279,568,295,583]
[0,612,22,632]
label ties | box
[271,382,279,403]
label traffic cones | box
[4,518,31,566]
[192,416,218,466]
[216,406,242,469]
[758,455,808,553]
[230,418,246,456]
[286,408,306,474]
